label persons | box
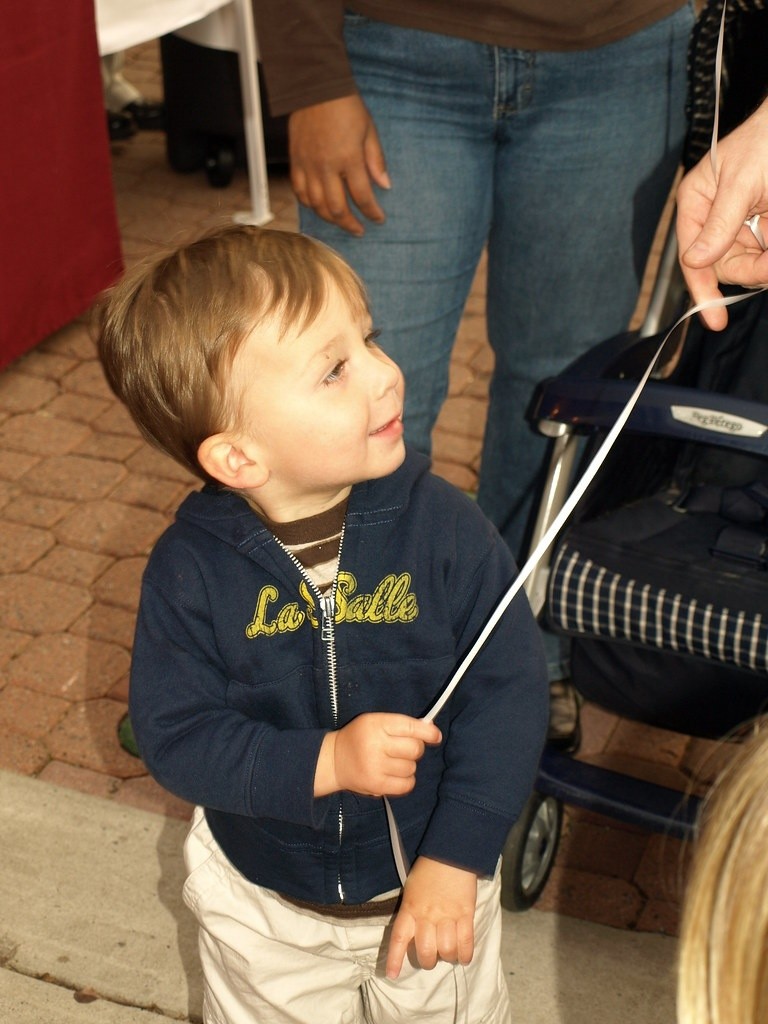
[254,2,700,677]
[92,229,552,1024]
[672,96,768,329]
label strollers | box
[502,0,767,911]
